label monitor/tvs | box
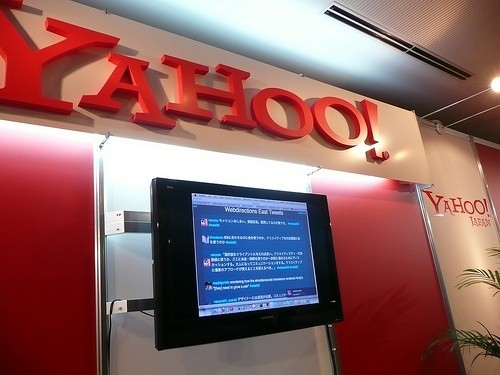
[151,178,344,351]
